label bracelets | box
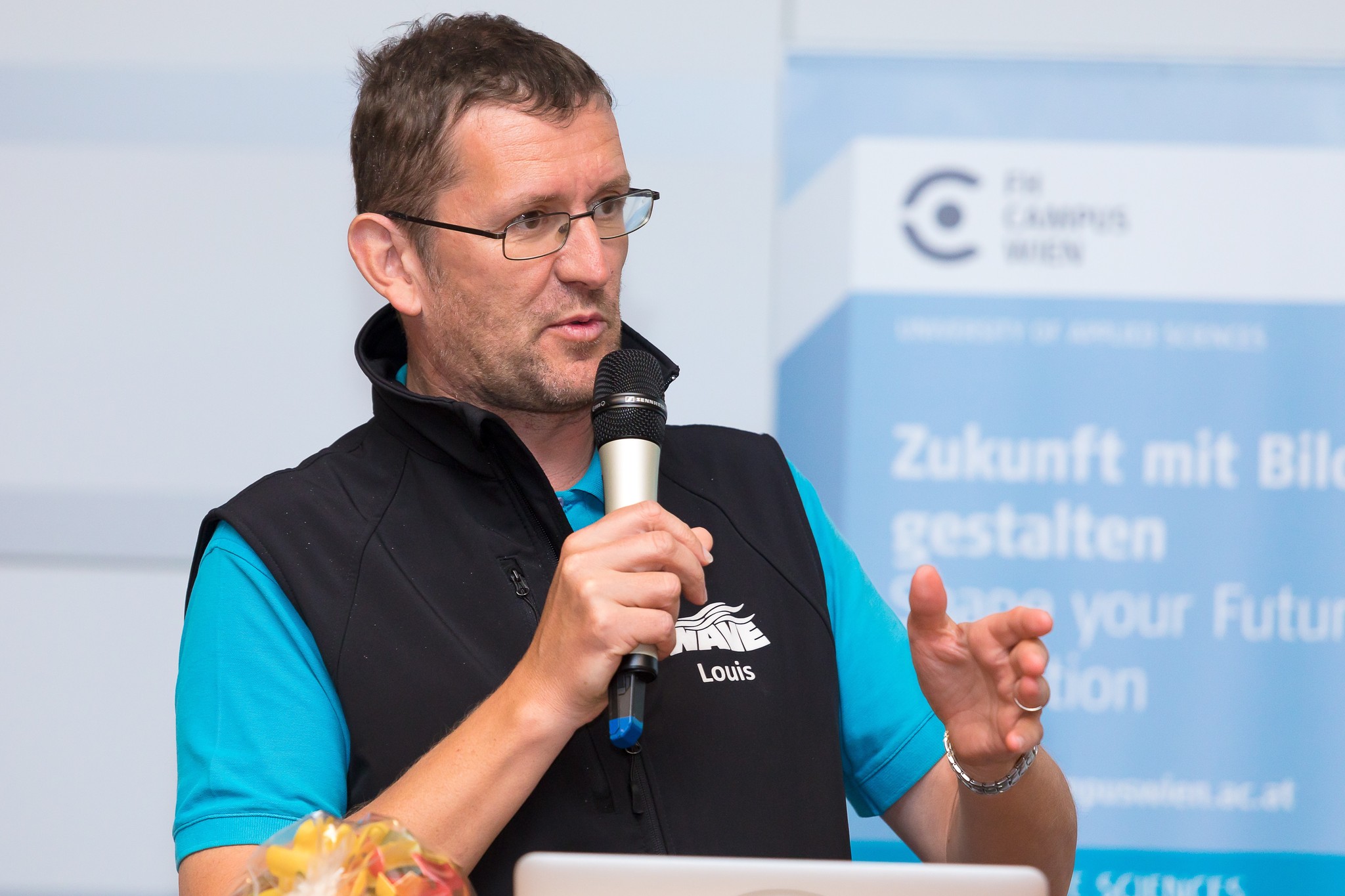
[942,729,1040,796]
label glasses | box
[385,188,661,262]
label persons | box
[169,13,1078,896]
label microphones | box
[591,347,668,751]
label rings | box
[1013,684,1044,712]
